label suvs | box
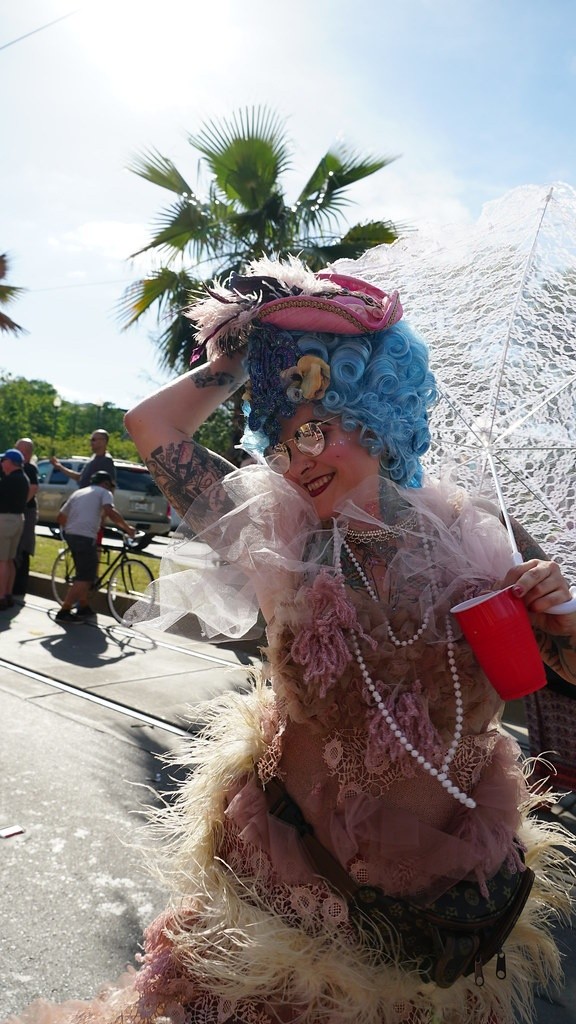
[30,451,171,550]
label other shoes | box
[12,595,26,607]
[5,594,15,607]
[0,597,8,610]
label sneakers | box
[76,605,96,618]
[55,612,86,625]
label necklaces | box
[336,512,436,646]
[331,526,478,809]
[336,507,420,546]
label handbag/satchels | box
[346,832,536,989]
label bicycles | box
[51,512,155,624]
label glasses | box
[89,437,103,441]
[263,413,342,474]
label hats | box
[0,449,26,465]
[254,273,404,336]
[94,471,116,488]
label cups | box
[449,586,547,701]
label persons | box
[14,438,40,594]
[49,428,117,587]
[58,469,137,623]
[0,448,30,610]
[123,310,575,1023]
